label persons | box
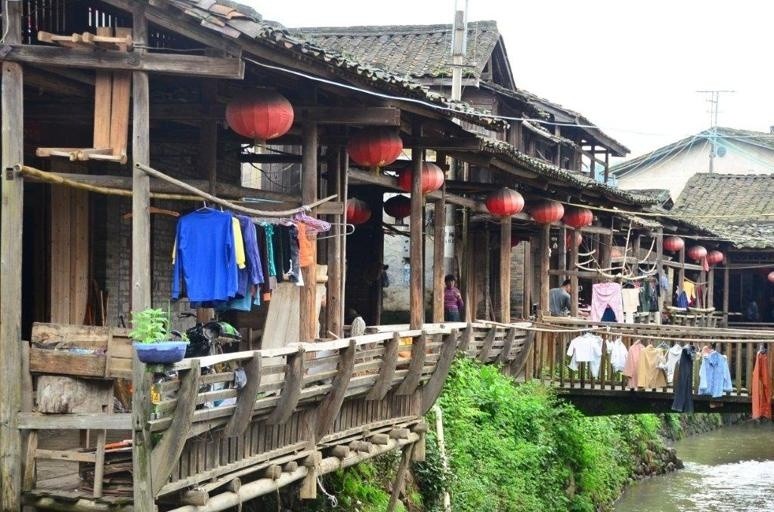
[548,279,590,319]
[441,275,464,321]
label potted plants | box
[129,307,191,363]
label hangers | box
[591,273,697,289]
[567,331,769,359]
[176,195,356,240]
